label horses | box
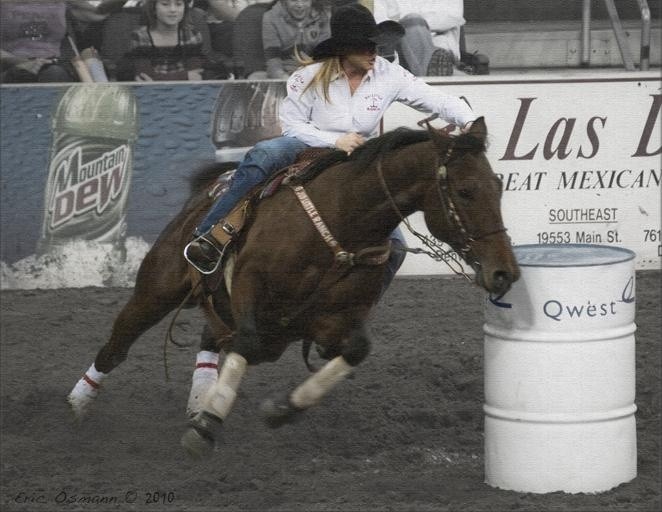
[62,115,522,458]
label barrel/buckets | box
[482,244,639,497]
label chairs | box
[95,3,274,81]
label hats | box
[313,4,404,60]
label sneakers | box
[187,238,220,261]
[426,48,453,75]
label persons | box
[1,0,125,85]
[126,1,209,82]
[374,0,464,62]
[184,0,490,266]
[262,0,332,79]
[202,0,267,81]
